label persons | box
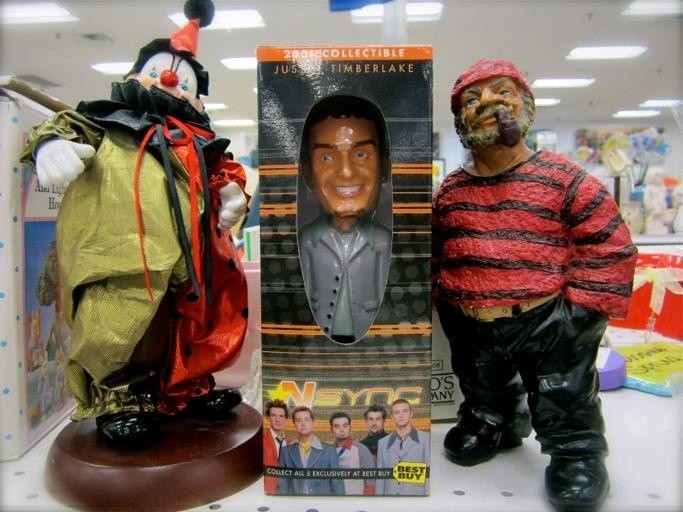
[296,96,392,344]
[433,57,640,511]
[262,399,431,496]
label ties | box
[275,437,285,464]
